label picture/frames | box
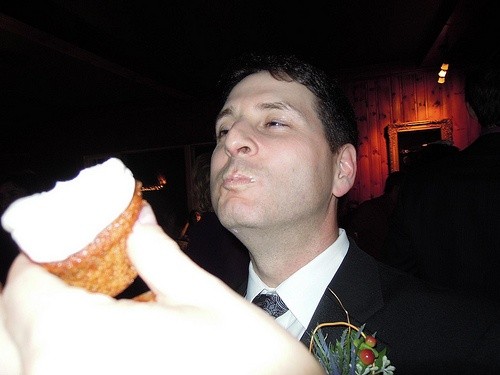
[385,118,454,174]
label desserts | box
[0,157,143,298]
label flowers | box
[309,323,395,375]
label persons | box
[0,54,441,375]
[380,69,500,304]
[0,125,404,293]
